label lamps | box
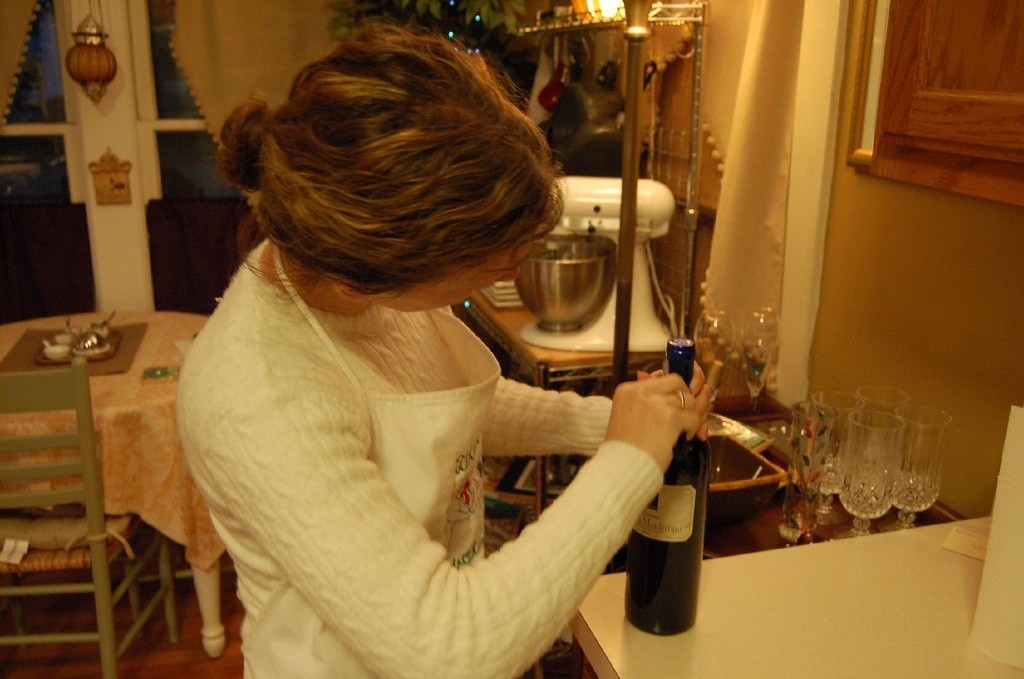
[64,12,116,101]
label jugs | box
[72,328,106,350]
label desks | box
[0,312,227,657]
[568,516,1024,679]
[549,395,964,576]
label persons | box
[176,21,710,678]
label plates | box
[72,343,110,356]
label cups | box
[856,385,910,421]
[778,401,838,541]
[811,389,865,496]
[838,410,906,520]
[892,404,953,512]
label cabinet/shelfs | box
[442,291,705,679]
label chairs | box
[0,357,179,679]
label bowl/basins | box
[91,326,109,339]
[516,236,616,333]
[44,345,71,360]
[54,334,72,345]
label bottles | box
[624,338,710,635]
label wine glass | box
[695,310,733,413]
[741,306,781,417]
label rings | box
[675,389,685,411]
